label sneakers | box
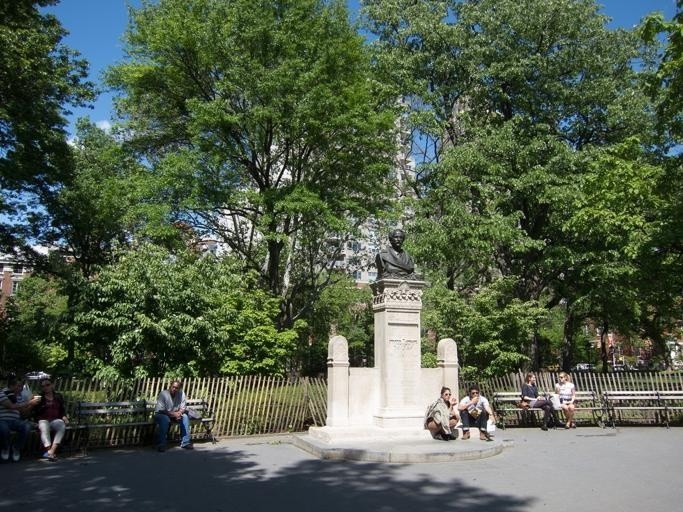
[460,430,470,440]
[157,442,194,453]
[540,420,578,431]
[479,431,492,441]
[0,448,21,462]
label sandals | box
[42,450,59,461]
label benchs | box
[492,390,683,426]
[28,399,216,456]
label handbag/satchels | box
[188,407,203,420]
[516,401,531,410]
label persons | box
[521,372,566,430]
[31,377,69,461]
[375,228,421,280]
[554,371,577,429]
[456,385,496,440]
[152,381,194,452]
[422,386,460,441]
[0,374,40,462]
